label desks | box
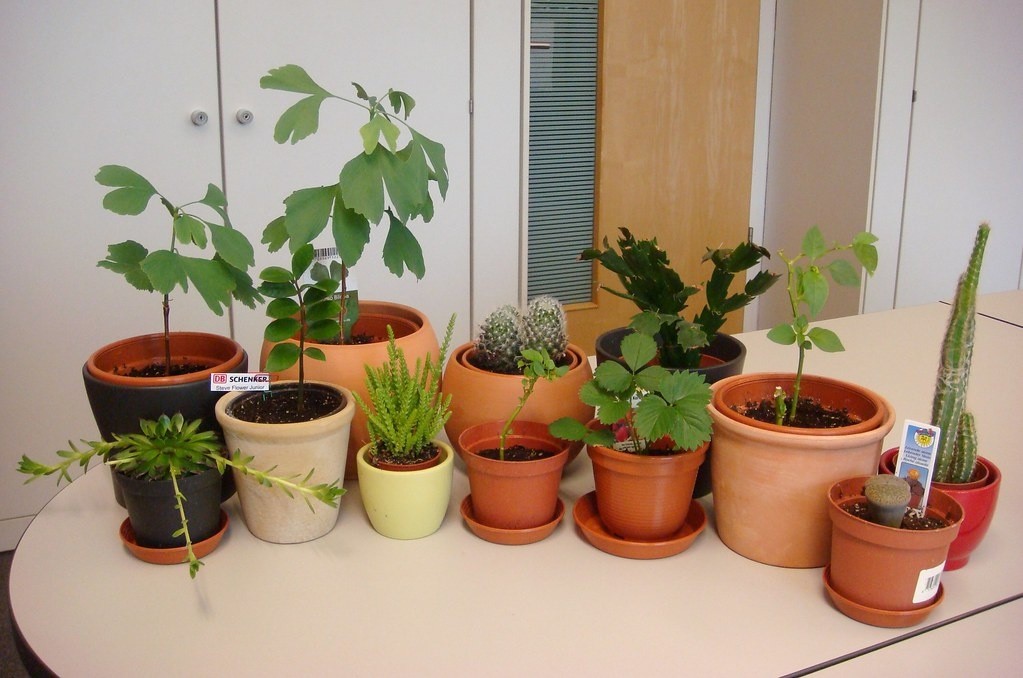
[10,289,1023,678]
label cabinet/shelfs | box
[0,0,472,536]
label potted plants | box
[258,65,448,481]
[215,244,355,544]
[458,347,572,530]
[706,226,896,568]
[82,165,266,509]
[109,412,220,547]
[575,225,782,498]
[821,475,964,611]
[441,296,596,465]
[350,311,456,539]
[880,221,1001,573]
[546,333,714,539]
